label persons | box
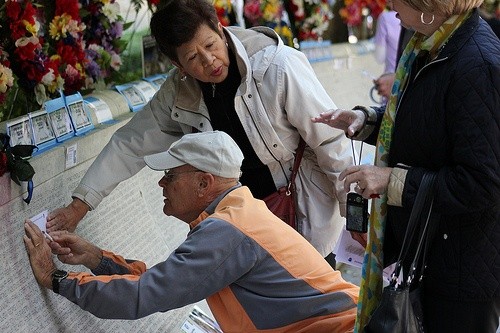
[46,0,368,271]
[23,130,360,333]
[310,0,500,333]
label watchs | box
[51,269,69,293]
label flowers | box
[0,0,386,122]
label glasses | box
[164,168,207,182]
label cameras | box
[346,192,368,233]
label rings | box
[35,243,41,246]
[356,185,365,190]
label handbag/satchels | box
[263,184,298,229]
[364,170,440,333]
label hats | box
[142,130,244,178]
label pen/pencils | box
[42,230,53,243]
